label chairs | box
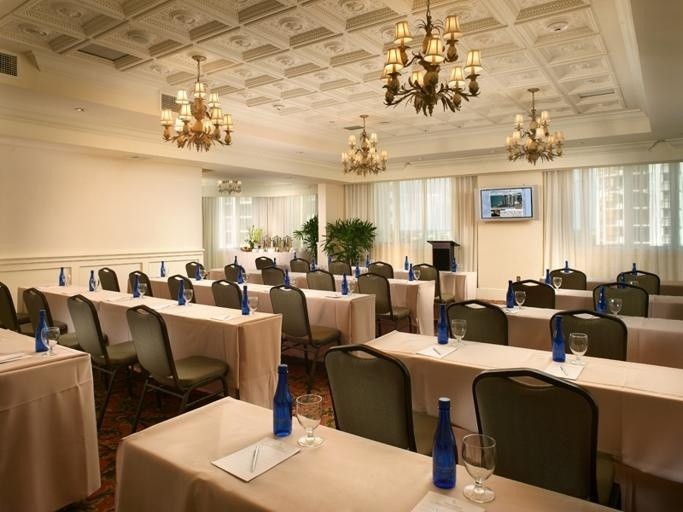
[592,282,649,318]
[549,308,628,362]
[446,299,508,346]
[356,273,412,336]
[261,266,286,287]
[269,285,342,395]
[98,268,120,292]
[255,256,274,268]
[15,308,31,324]
[367,261,394,279]
[616,270,661,296]
[409,264,455,321]
[290,259,310,272]
[0,281,66,339]
[167,275,197,304]
[328,260,353,276]
[548,268,587,291]
[23,286,109,349]
[211,279,243,309]
[124,304,231,434]
[511,279,556,310]
[128,270,154,298]
[323,344,416,452]
[66,293,140,429]
[186,261,205,278]
[468,365,599,503]
[306,269,336,291]
[224,264,247,283]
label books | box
[212,438,301,483]
[410,492,513,511]
[0,351,36,365]
[544,361,584,380]
[33,283,243,324]
[416,345,457,359]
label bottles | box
[270,362,292,438]
[34,309,53,353]
[431,395,457,490]
[506,260,569,308]
[353,254,414,282]
[595,262,636,312]
[57,265,249,316]
[550,311,567,363]
[158,251,349,294]
[436,301,449,344]
[451,257,455,273]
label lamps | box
[381,0,485,117]
[341,114,388,178]
[216,178,243,194]
[161,54,235,152]
[506,88,564,165]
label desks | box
[351,264,477,303]
[498,301,683,367]
[142,274,378,350]
[554,288,683,319]
[210,267,436,335]
[0,326,104,510]
[17,282,285,409]
[232,247,294,268]
[361,330,683,482]
[107,396,612,512]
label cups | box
[607,296,623,319]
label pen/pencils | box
[252,445,259,472]
[560,366,568,376]
[433,348,440,355]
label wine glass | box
[245,295,261,316]
[413,270,420,282]
[295,393,328,448]
[449,317,469,346]
[345,278,356,293]
[40,327,61,356]
[461,430,496,504]
[567,332,587,364]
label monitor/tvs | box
[479,185,534,220]
